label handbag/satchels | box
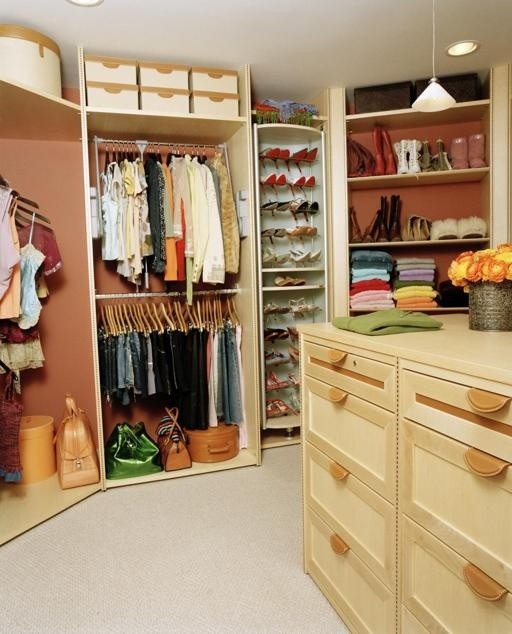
[154,406,193,472]
[51,392,101,491]
[104,421,165,481]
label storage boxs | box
[3,414,54,486]
[182,421,241,464]
[1,25,65,99]
[85,54,242,120]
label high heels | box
[300,199,319,222]
[270,149,290,169]
[298,225,318,247]
[268,199,290,217]
[260,227,275,246]
[263,297,323,420]
[285,174,306,198]
[284,148,308,172]
[270,172,287,196]
[259,147,280,169]
[259,172,276,196]
[260,200,278,217]
[267,228,287,245]
[289,199,309,222]
[298,174,316,199]
[294,147,319,173]
[285,225,307,245]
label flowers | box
[447,240,512,296]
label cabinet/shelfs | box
[397,349,512,633]
[250,122,332,434]
[342,98,492,314]
[284,314,398,633]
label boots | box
[347,205,363,244]
[401,210,433,241]
[347,139,364,178]
[408,137,423,175]
[381,125,398,176]
[388,194,404,243]
[432,137,453,172]
[376,192,389,242]
[356,137,375,178]
[418,139,436,174]
[449,134,469,170]
[468,131,488,169]
[371,125,387,175]
[393,137,410,175]
[412,217,433,241]
[362,206,382,244]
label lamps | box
[411,1,457,114]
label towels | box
[330,308,445,333]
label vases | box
[467,276,512,332]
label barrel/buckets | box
[469,282,512,330]
[16,415,56,484]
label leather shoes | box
[287,248,311,264]
[295,248,323,263]
[273,273,292,287]
[284,274,306,286]
[275,252,291,265]
[262,248,277,265]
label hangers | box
[103,136,224,176]
[1,172,53,236]
[91,288,243,339]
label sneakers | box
[428,217,460,241]
[459,213,489,239]
